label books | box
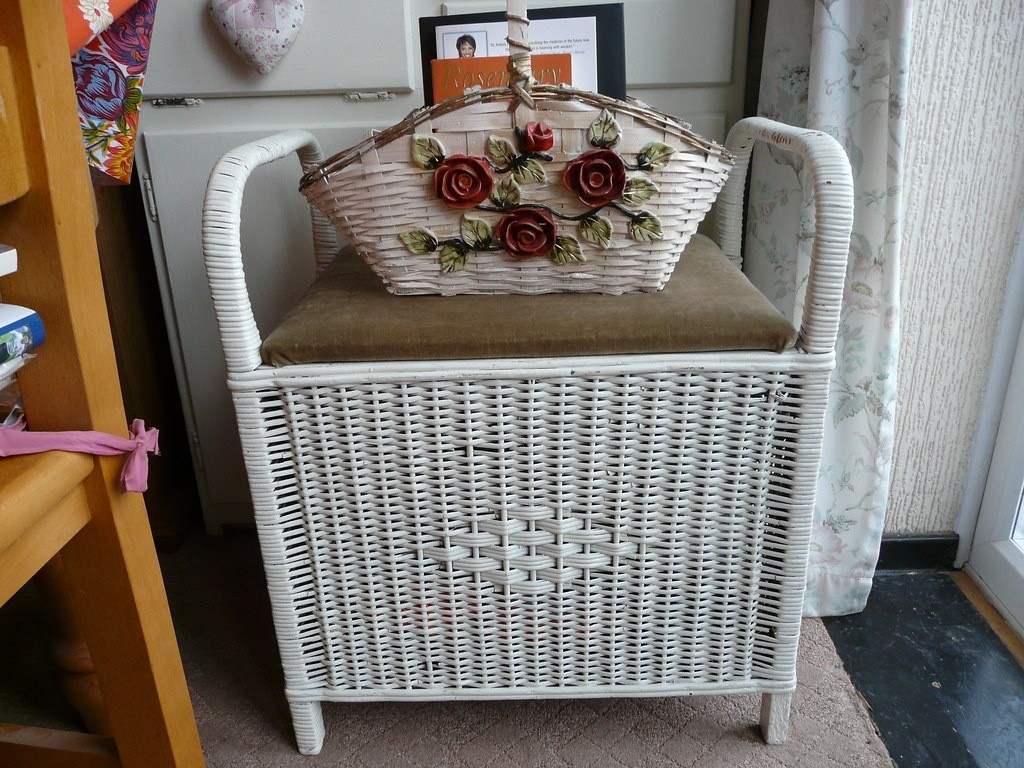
[0,243,17,276]
[1,303,46,362]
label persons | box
[456,34,478,59]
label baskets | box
[297,0,736,297]
